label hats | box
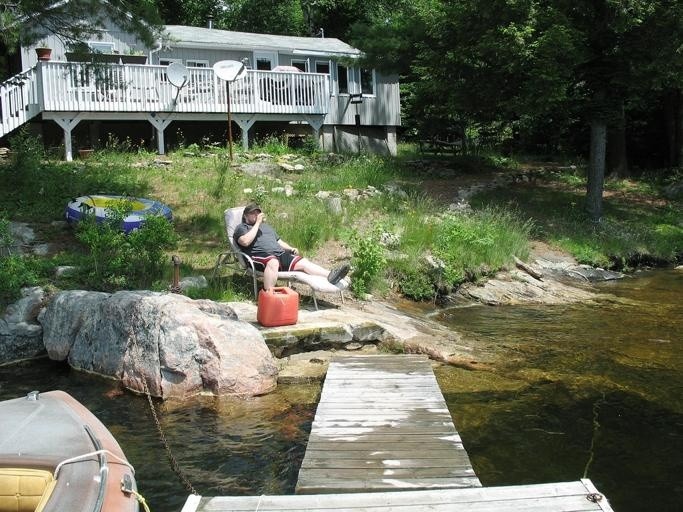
[243,204,263,214]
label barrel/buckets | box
[257,286,299,327]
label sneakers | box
[328,262,349,285]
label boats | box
[66,192,174,240]
[1,390,141,512]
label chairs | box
[214,206,353,311]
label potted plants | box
[36,41,53,65]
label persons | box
[233,205,349,291]
